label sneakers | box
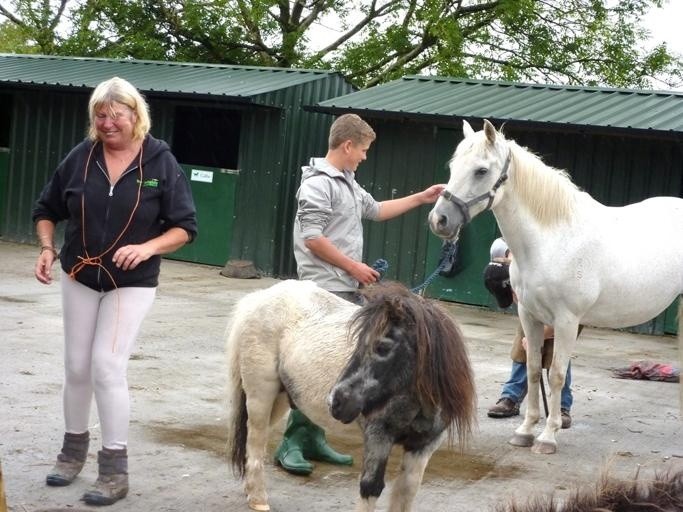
[488,398,519,417]
[561,408,571,427]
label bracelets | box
[40,246,57,260]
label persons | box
[32,76,199,506]
[274,112,449,476]
[490,236,512,261]
[483,257,584,429]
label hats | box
[484,262,513,308]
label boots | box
[46,432,89,486]
[273,409,313,474]
[84,446,128,505]
[303,420,352,465]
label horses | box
[228,277,475,512]
[428,118,683,456]
